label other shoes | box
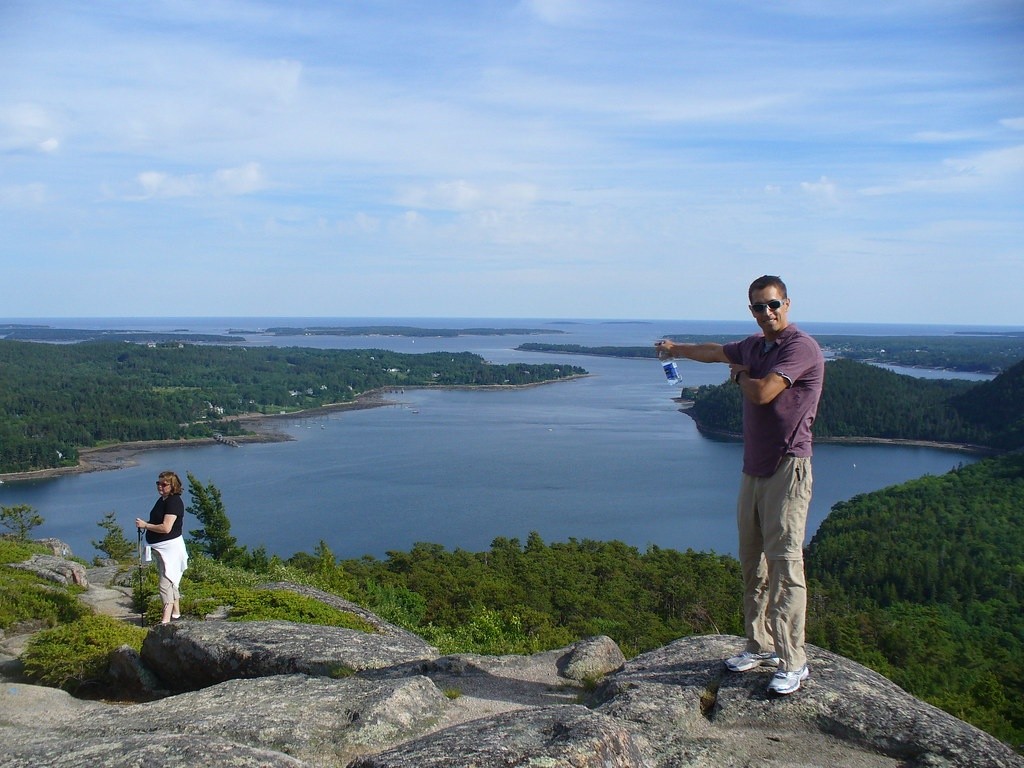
[172,614,181,619]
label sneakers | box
[724,650,779,671]
[767,663,809,694]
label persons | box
[136,472,189,624]
[654,276,825,695]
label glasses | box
[156,481,171,487]
[752,299,784,313]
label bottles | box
[659,342,683,386]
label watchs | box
[734,370,746,385]
